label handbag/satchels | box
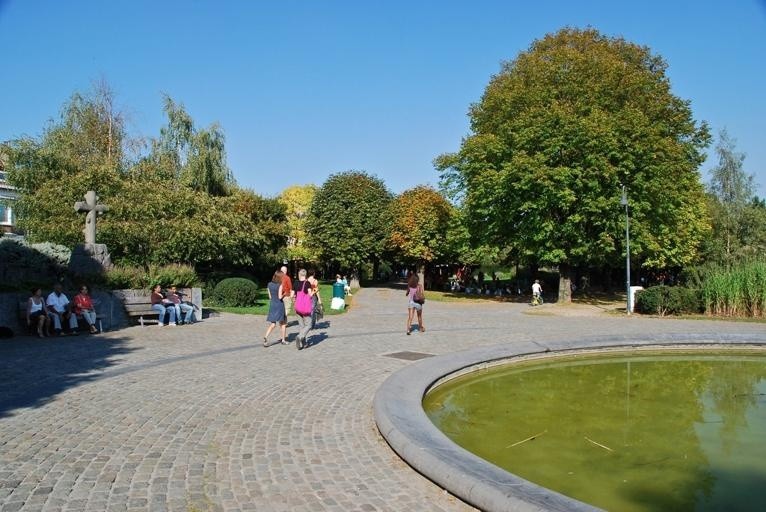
[160,298,175,308]
[283,296,295,316]
[413,291,425,305]
[295,291,313,315]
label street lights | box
[619,186,631,316]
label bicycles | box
[531,290,543,306]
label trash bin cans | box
[333,283,344,300]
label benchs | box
[123,298,186,327]
[18,301,106,336]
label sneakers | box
[263,334,308,350]
[407,327,425,335]
[37,329,81,339]
[90,327,98,334]
[158,321,192,327]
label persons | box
[166,285,194,325]
[151,284,177,327]
[425,272,432,290]
[405,273,426,335]
[262,266,353,350]
[531,279,543,305]
[26,287,52,338]
[73,285,98,334]
[45,284,81,337]
